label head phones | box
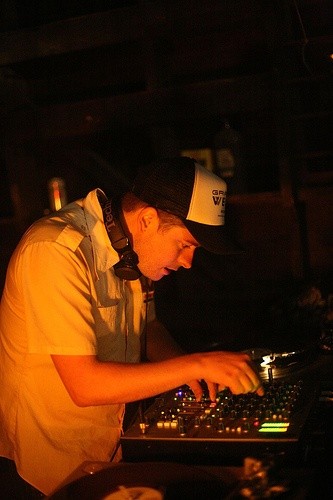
[102,193,143,280]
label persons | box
[0,157,265,500]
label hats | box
[124,155,240,256]
